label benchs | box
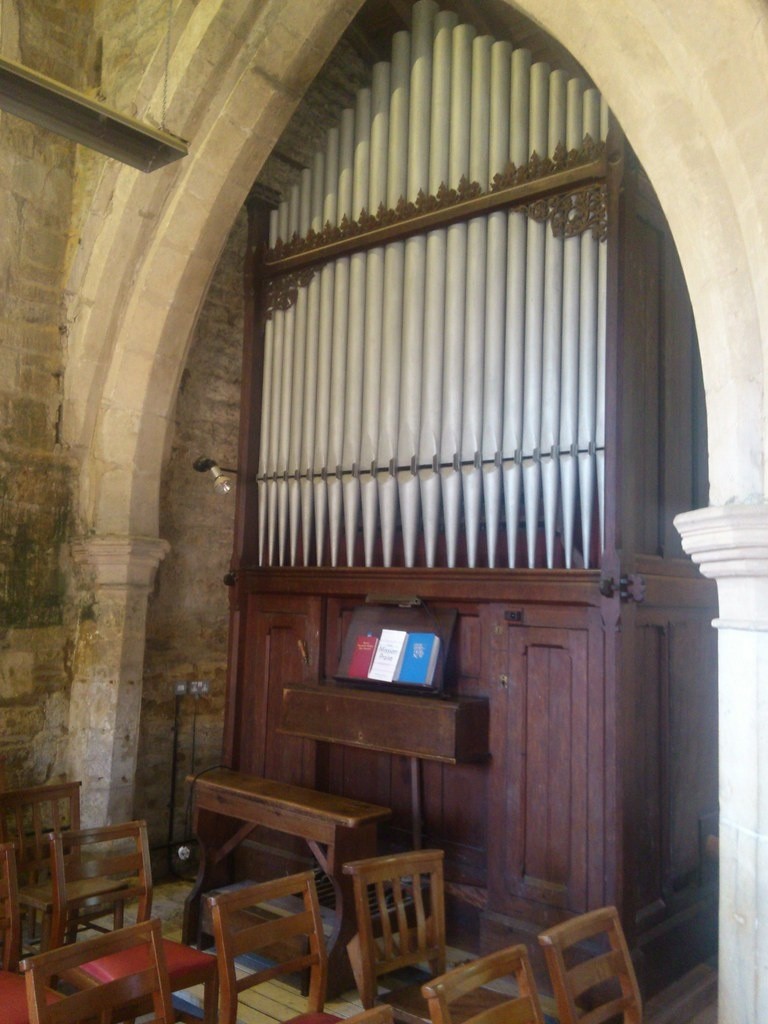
[182,768,390,999]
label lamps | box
[194,454,237,493]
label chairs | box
[345,852,515,1024]
[0,841,69,1024]
[421,943,544,1024]
[205,872,344,1024]
[47,821,217,1024]
[0,782,127,984]
[538,905,647,1024]
[19,918,179,1023]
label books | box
[347,628,441,687]
